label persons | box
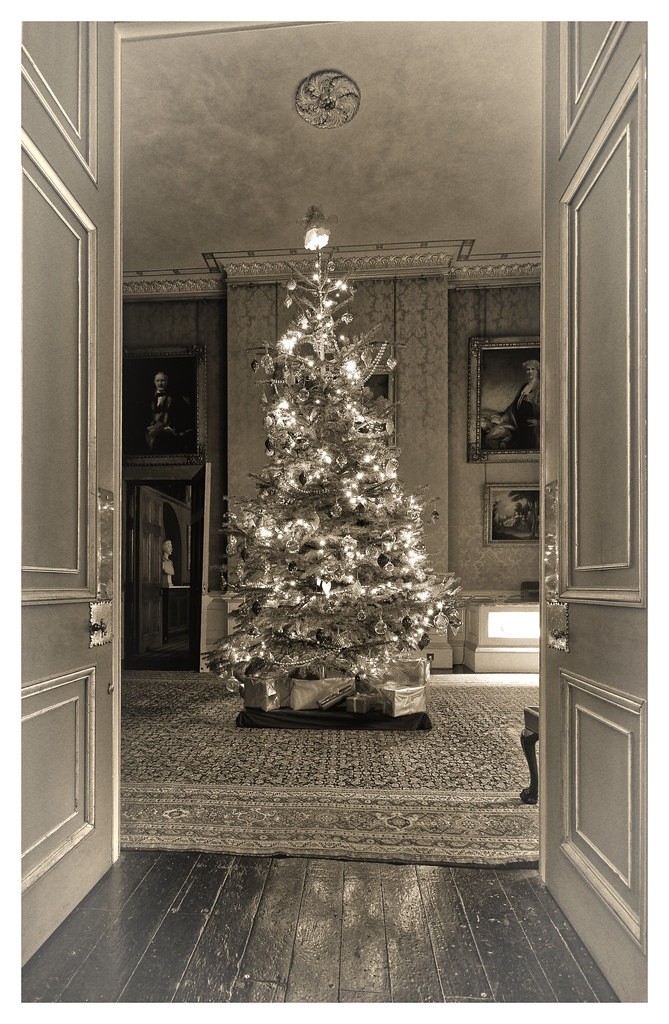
[162,539,175,575]
[488,360,540,449]
[143,369,179,452]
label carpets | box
[118,668,543,869]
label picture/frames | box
[482,482,540,548]
[465,334,540,463]
[120,342,207,466]
[274,365,398,450]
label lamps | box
[302,207,331,250]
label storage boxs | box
[346,694,372,714]
